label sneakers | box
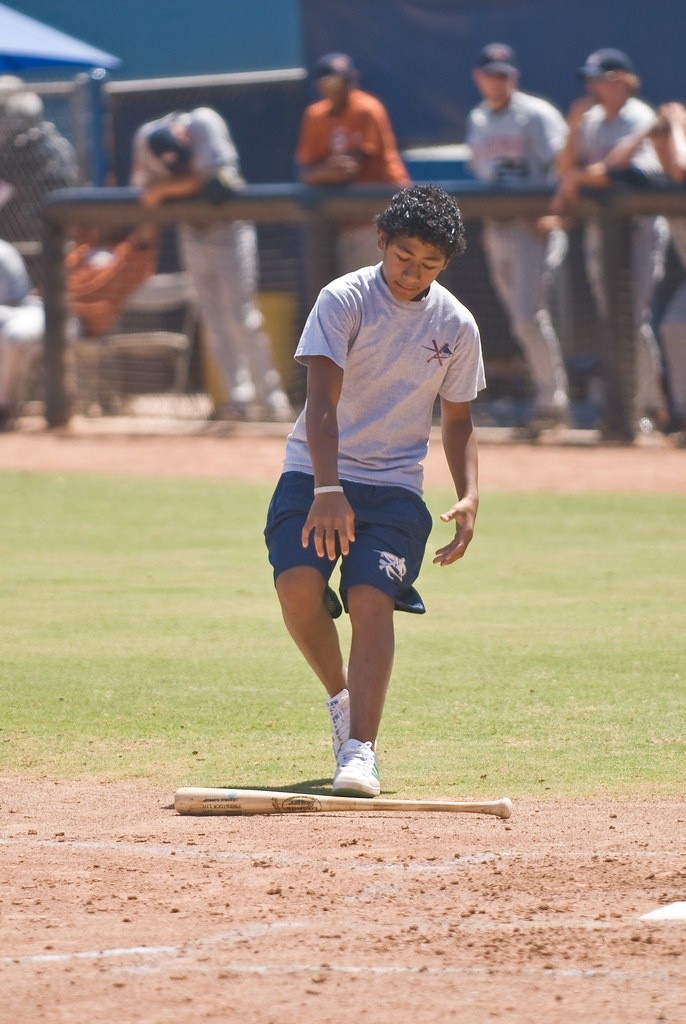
[333,739,381,797]
[326,688,350,759]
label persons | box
[297,52,411,275]
[131,107,295,422]
[469,43,569,431]
[0,74,77,430]
[560,47,686,444]
[264,186,486,799]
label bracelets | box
[314,486,343,496]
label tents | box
[0,0,122,187]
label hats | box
[312,52,349,79]
[476,43,519,76]
[147,128,190,172]
[578,50,633,80]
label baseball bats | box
[175,786,512,817]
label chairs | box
[109,270,195,393]
